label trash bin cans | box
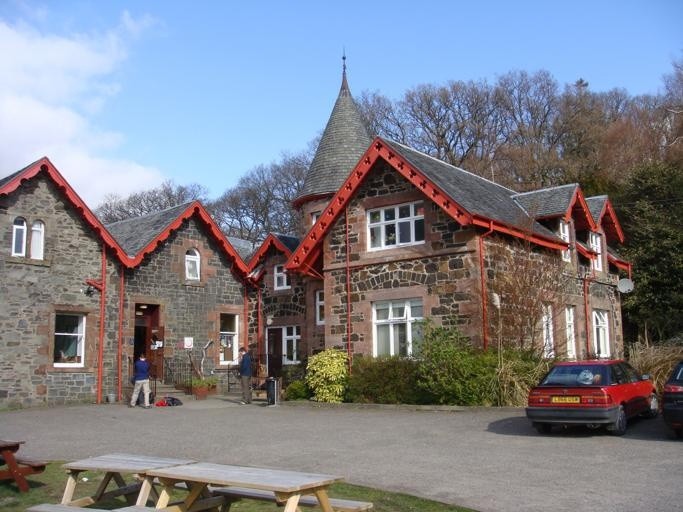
[265,376,282,406]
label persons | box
[238,348,253,405]
[128,353,153,408]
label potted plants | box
[183,376,219,400]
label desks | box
[59,447,346,511]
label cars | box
[525,359,659,436]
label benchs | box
[24,501,111,512]
[130,473,374,511]
